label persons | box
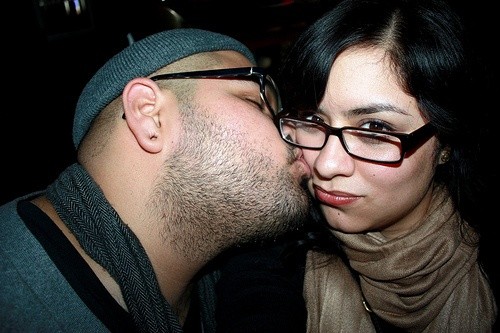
[0,22,311,332]
[214,2,500,333]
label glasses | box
[273,106,456,164]
[121,66,284,121]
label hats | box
[72,28,257,153]
